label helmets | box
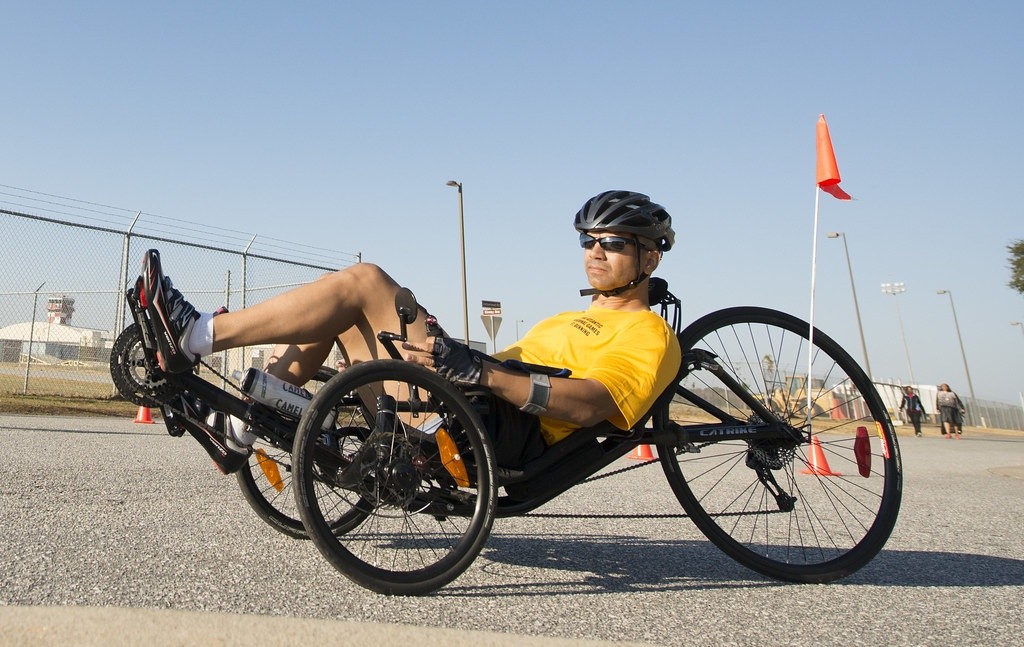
[572,190,676,253]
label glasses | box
[577,234,655,254]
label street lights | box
[446,179,472,350]
[878,279,916,387]
[1010,321,1024,339]
[825,231,873,378]
[934,288,979,426]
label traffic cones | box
[131,405,158,425]
[800,436,843,477]
[628,439,656,463]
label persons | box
[135,191,681,485]
[936,384,965,439]
[898,386,925,437]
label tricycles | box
[110,275,906,601]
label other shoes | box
[955,434,959,440]
[945,433,951,439]
[916,433,922,437]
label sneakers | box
[172,393,255,476]
[141,250,201,374]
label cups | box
[241,368,338,435]
